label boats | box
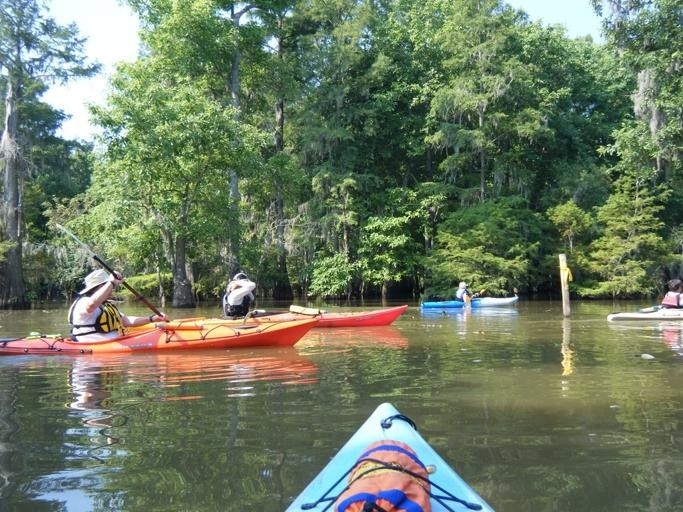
[607,305,683,321]
[420,293,520,309]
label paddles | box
[466,288,487,303]
[56,224,169,322]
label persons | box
[454,281,481,301]
[661,328,683,358]
[660,278,682,308]
[63,353,139,447]
[67,268,170,342]
[222,272,265,319]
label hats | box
[78,269,112,294]
[457,281,468,289]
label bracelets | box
[149,314,154,323]
